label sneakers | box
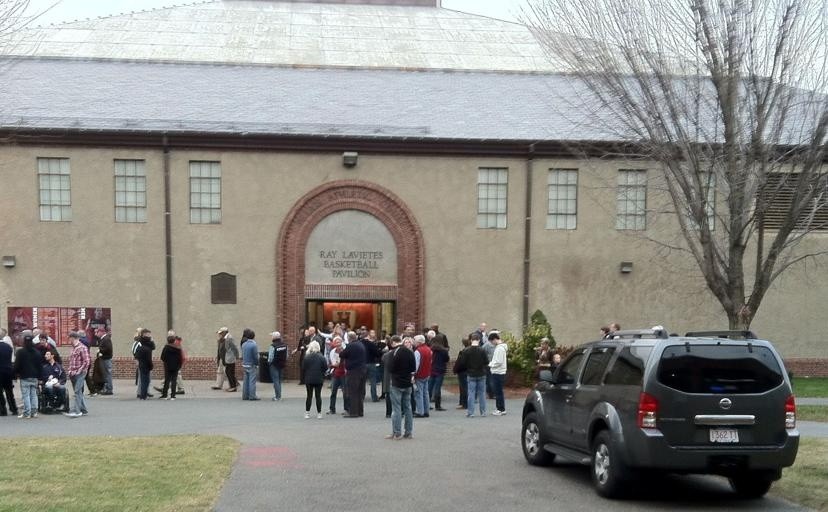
[384,432,403,439]
[317,413,322,418]
[326,409,336,414]
[342,410,349,414]
[492,409,507,416]
[402,431,412,439]
[159,396,167,400]
[171,396,177,400]
[66,410,81,417]
[303,411,311,417]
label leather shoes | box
[152,386,165,392]
[147,392,153,396]
[343,413,358,417]
[226,387,237,392]
[211,385,221,389]
[17,413,30,418]
[173,390,185,394]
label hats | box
[269,331,280,336]
[39,334,47,340]
[78,330,87,336]
[218,327,228,333]
[21,330,32,337]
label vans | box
[520,326,800,499]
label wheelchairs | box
[37,379,69,413]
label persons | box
[77,330,91,396]
[88,327,104,396]
[268,331,286,401]
[609,323,622,339]
[551,354,561,374]
[358,326,368,341]
[299,327,306,338]
[359,332,380,402]
[297,329,308,385]
[473,324,489,345]
[159,336,183,399]
[14,330,42,418]
[425,330,436,347]
[463,334,490,416]
[218,328,240,392]
[412,335,432,416]
[482,329,501,399]
[328,337,347,414]
[34,333,61,365]
[96,328,114,394]
[32,327,56,349]
[403,326,415,337]
[0,328,15,389]
[533,339,555,383]
[323,320,351,337]
[599,326,610,340]
[380,340,395,418]
[242,331,261,401]
[339,331,367,417]
[211,332,241,390]
[132,327,153,398]
[387,335,417,440]
[453,338,468,409]
[487,333,508,415]
[133,328,156,400]
[430,334,450,411]
[400,337,414,351]
[241,328,252,347]
[367,330,377,344]
[43,352,67,407]
[0,329,19,416]
[302,326,324,360]
[378,329,391,348]
[153,337,188,394]
[301,340,327,419]
[432,324,449,346]
[64,333,90,417]
